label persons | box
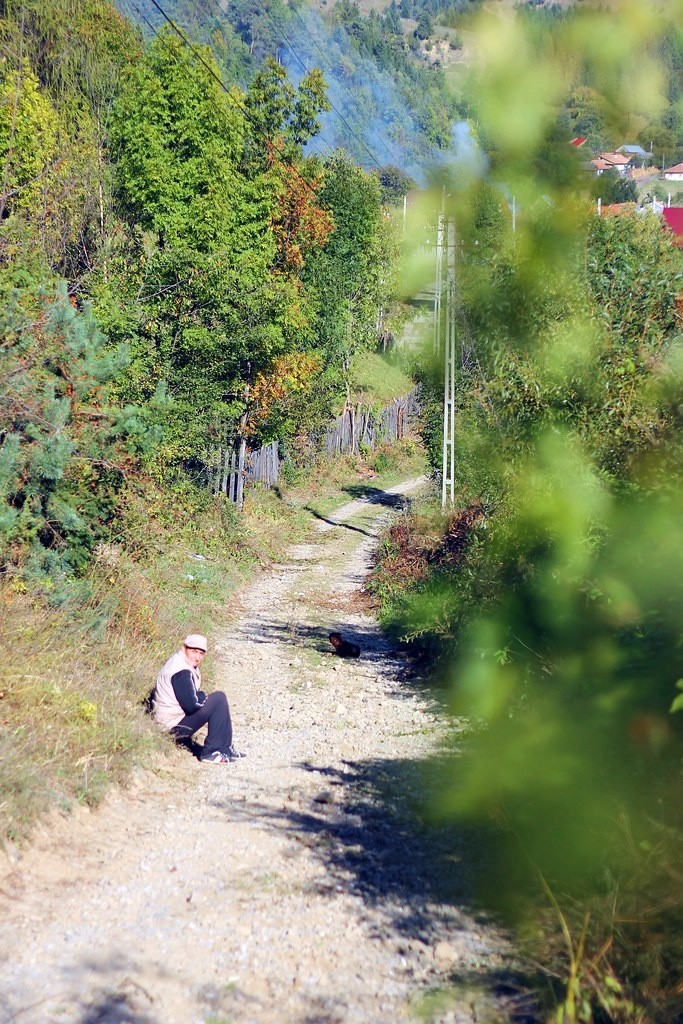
[153,634,248,764]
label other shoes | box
[230,745,247,759]
[200,749,237,765]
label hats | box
[183,633,208,651]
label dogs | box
[328,632,360,658]
